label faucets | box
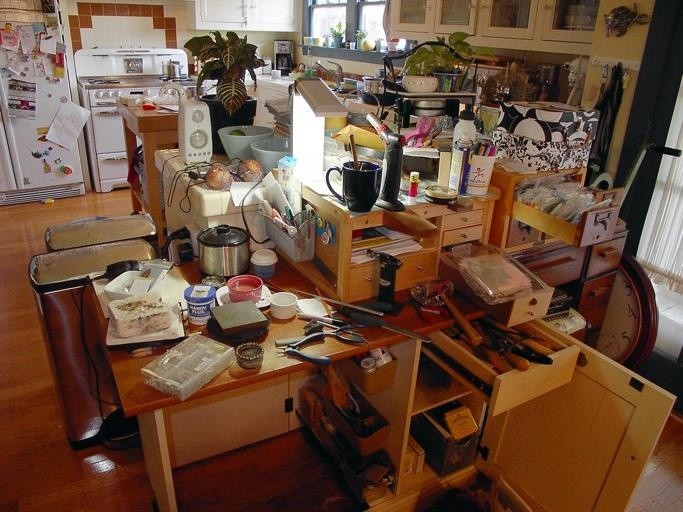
[316,59,343,85]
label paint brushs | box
[131,332,202,358]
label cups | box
[326,160,384,213]
[234,343,266,371]
[302,36,319,47]
[183,249,298,325]
[349,42,356,50]
[362,77,379,95]
[271,69,282,79]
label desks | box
[115,96,178,248]
[81,163,677,512]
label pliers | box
[338,392,369,427]
[276,324,366,365]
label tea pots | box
[168,60,183,79]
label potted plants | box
[417,32,497,94]
[183,30,266,156]
[401,53,439,93]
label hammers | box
[423,279,483,345]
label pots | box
[198,225,252,278]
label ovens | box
[85,107,127,192]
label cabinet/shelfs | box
[388,0,436,44]
[435,0,542,53]
[537,0,601,56]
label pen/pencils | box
[475,142,496,156]
[315,287,333,318]
[297,313,348,326]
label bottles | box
[304,48,314,79]
[448,109,497,198]
[406,171,420,206]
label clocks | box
[591,251,659,374]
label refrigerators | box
[0,0,84,206]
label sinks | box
[333,90,356,99]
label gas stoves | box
[83,76,199,107]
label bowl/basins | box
[413,99,446,117]
[217,125,295,171]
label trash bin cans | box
[28,214,160,450]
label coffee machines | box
[539,64,561,104]
[272,40,294,80]
